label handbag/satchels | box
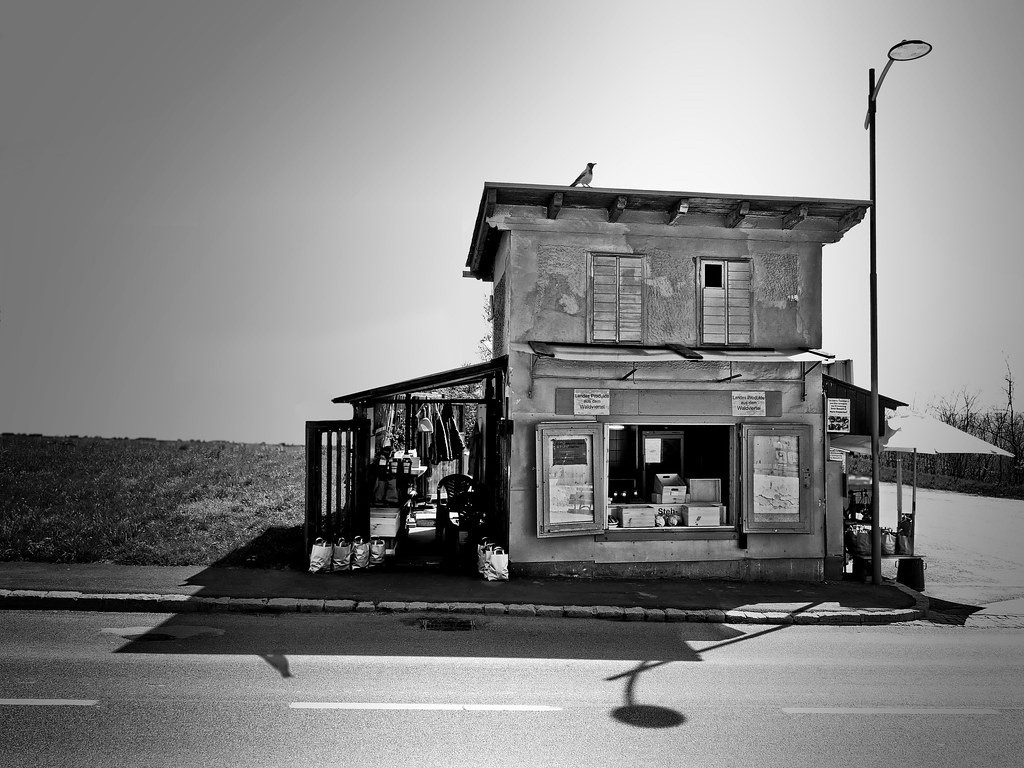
[477,537,510,581]
[849,523,912,556]
[307,535,387,574]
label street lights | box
[863,39,934,586]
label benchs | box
[569,487,593,514]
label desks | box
[387,466,427,477]
[860,553,927,560]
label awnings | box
[507,338,837,381]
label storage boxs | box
[682,505,720,526]
[415,513,436,527]
[653,473,687,503]
[370,507,401,537]
[403,457,421,468]
[617,506,655,528]
[684,479,722,503]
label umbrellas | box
[831,409,1014,557]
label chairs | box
[435,473,477,540]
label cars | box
[848,476,873,508]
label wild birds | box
[569,163,596,188]
